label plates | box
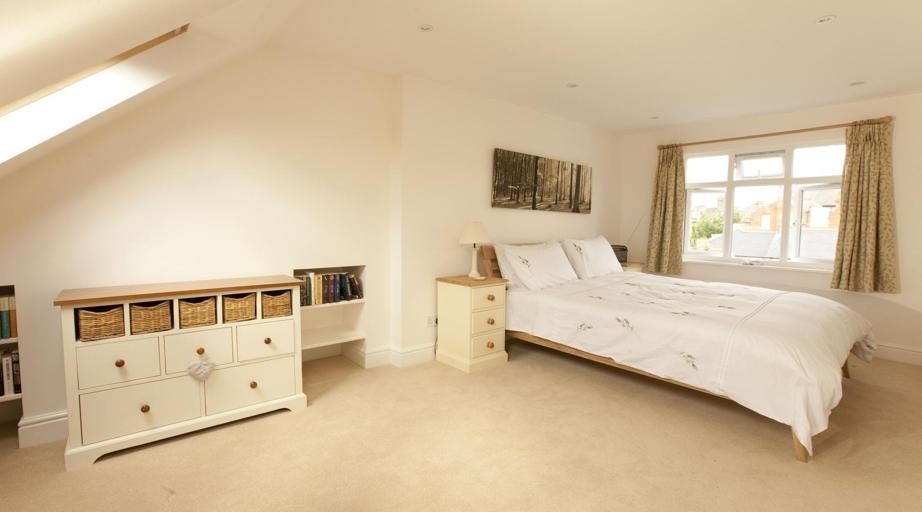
[51,273,307,472]
[293,265,367,353]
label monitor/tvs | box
[1,298,11,339]
[294,272,362,306]
[10,295,17,337]
[13,350,22,393]
[2,352,16,396]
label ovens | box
[479,241,852,464]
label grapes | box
[435,274,510,372]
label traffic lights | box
[494,236,625,289]
